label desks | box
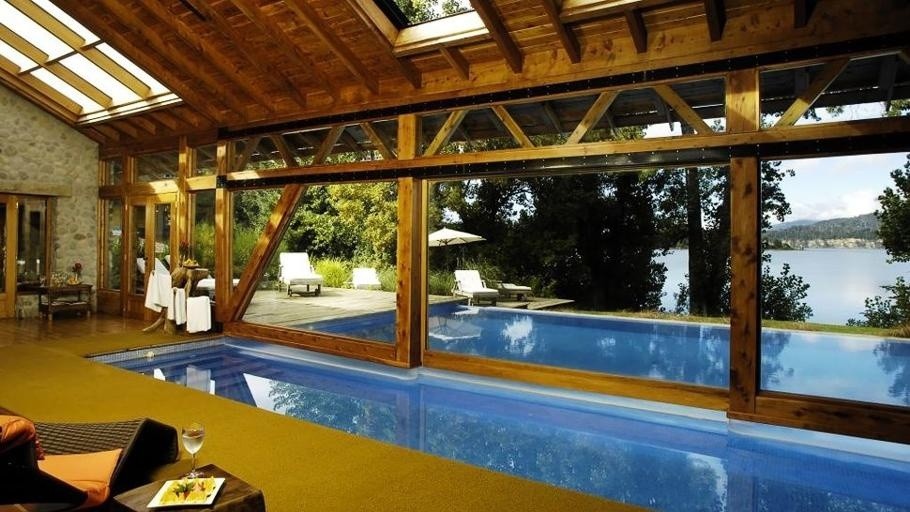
[33,283,95,322]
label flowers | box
[71,262,83,277]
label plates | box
[147,478,226,507]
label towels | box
[145,270,213,334]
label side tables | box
[111,462,267,511]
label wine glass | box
[182,422,205,478]
[39,274,45,288]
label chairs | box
[277,252,323,297]
[135,253,241,300]
[0,405,181,512]
[353,268,382,291]
[450,269,532,307]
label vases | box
[75,274,80,281]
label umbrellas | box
[428,226,487,271]
[428,316,485,342]
